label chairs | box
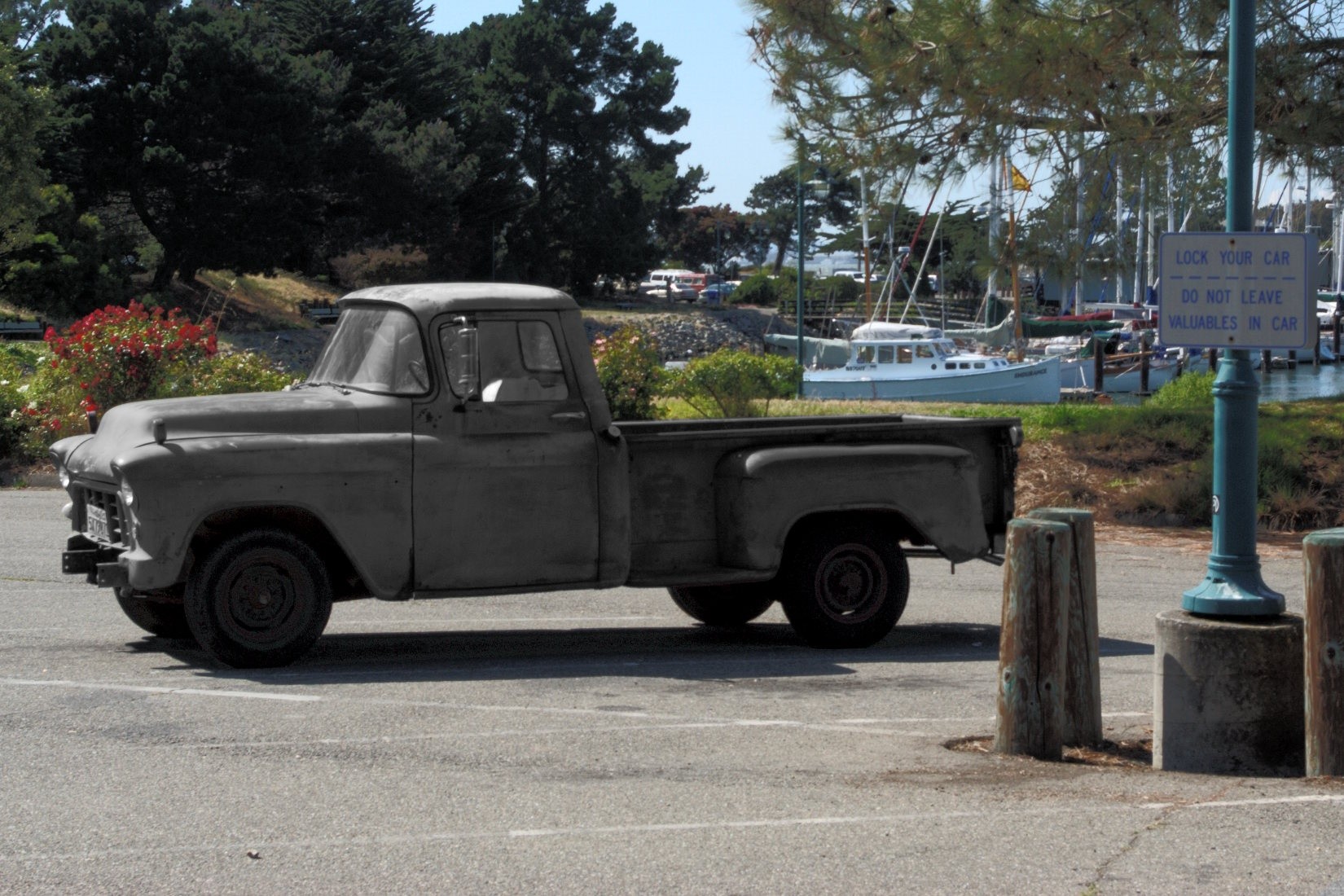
[482,377,527,403]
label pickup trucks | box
[47,280,1029,671]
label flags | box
[1003,156,1030,191]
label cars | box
[640,266,744,307]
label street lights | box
[716,219,731,307]
[795,133,832,400]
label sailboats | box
[760,80,1343,405]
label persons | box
[666,287,674,304]
[1002,342,1024,364]
